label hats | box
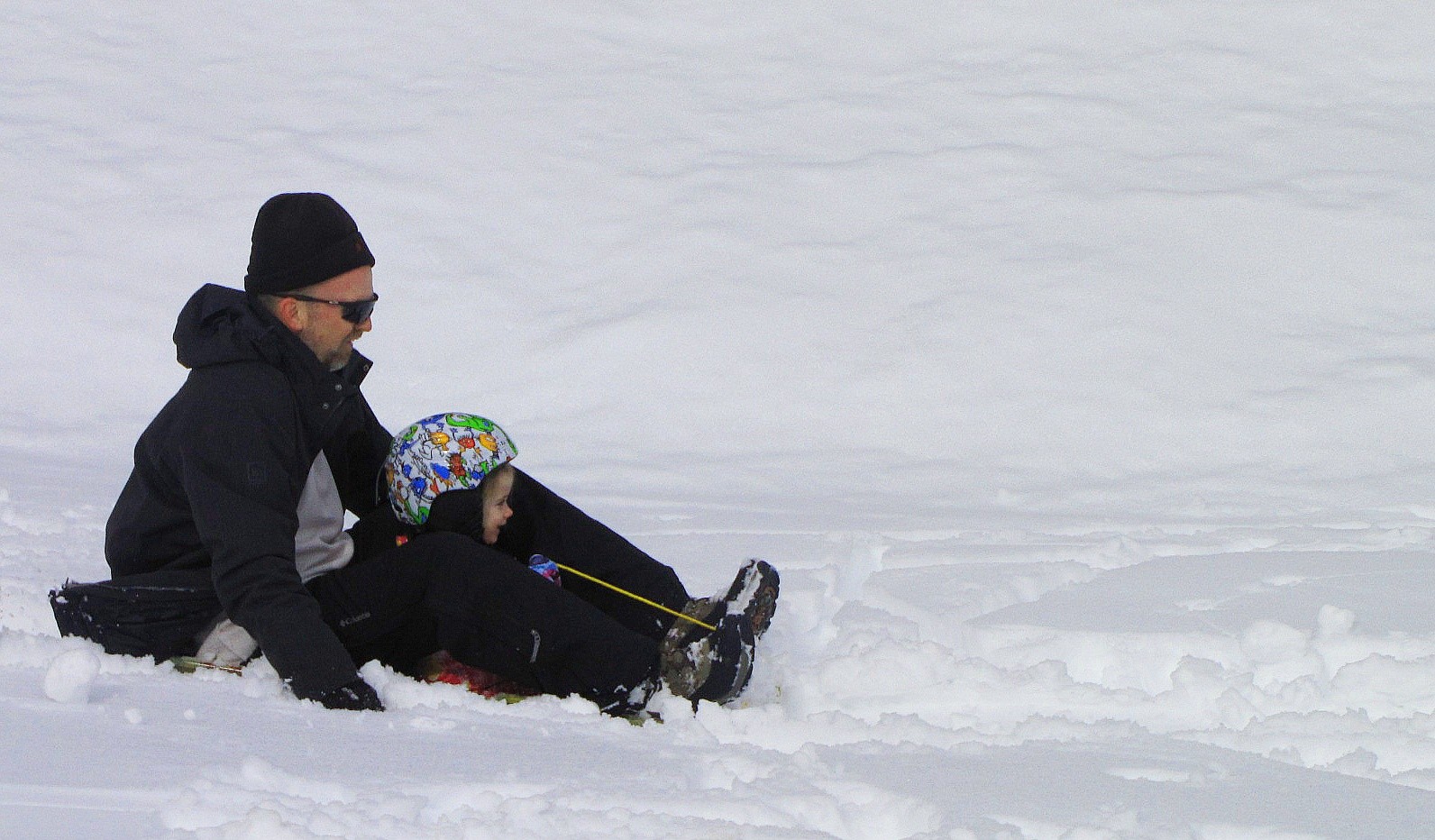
[244,192,376,295]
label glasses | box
[269,293,379,325]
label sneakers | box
[649,613,755,707]
[663,559,781,646]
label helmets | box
[384,412,519,536]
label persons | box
[100,193,780,714]
[380,412,561,707]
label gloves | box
[527,553,562,587]
[320,680,384,713]
